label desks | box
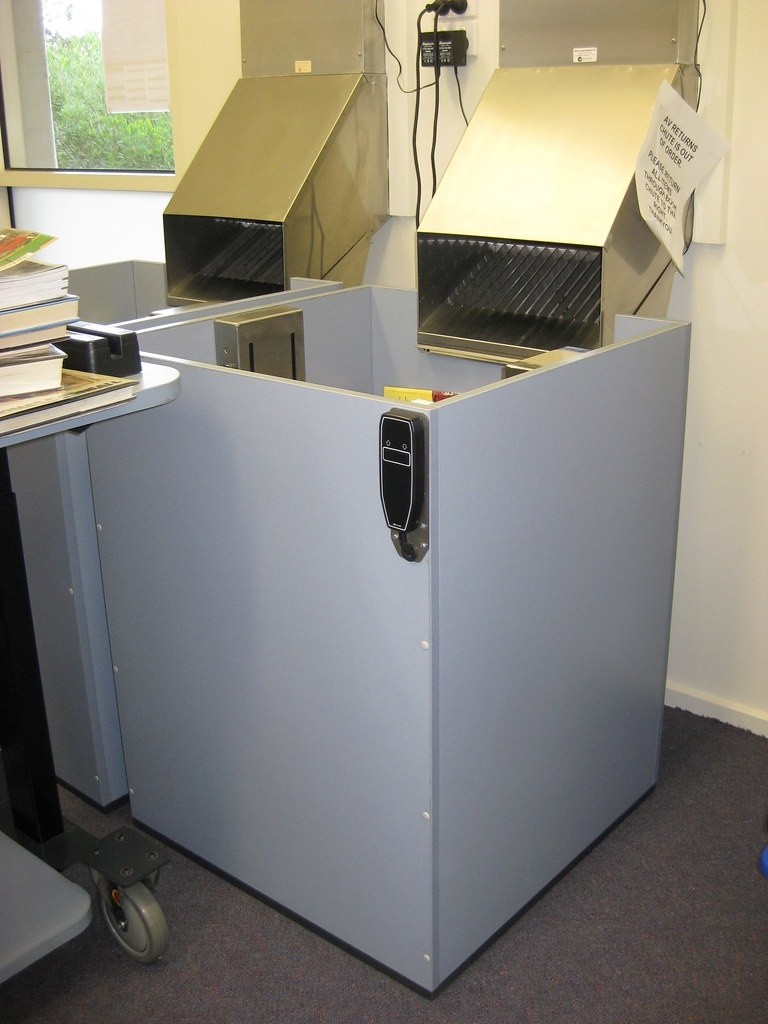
[0,363,180,845]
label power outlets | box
[437,0,477,20]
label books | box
[0,344,68,398]
[384,386,459,403]
[0,295,80,335]
[0,228,69,310]
[0,324,74,352]
[0,368,139,437]
